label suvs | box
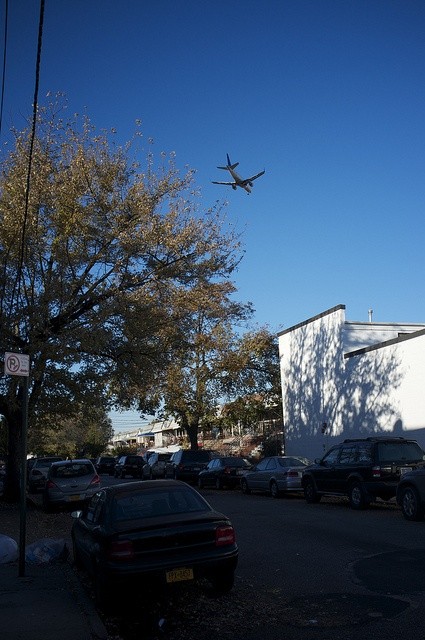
[165,449,212,481]
[300,437,425,510]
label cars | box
[46,457,101,502]
[114,455,146,479]
[29,458,64,497]
[395,471,425,520]
[96,457,117,475]
[198,457,254,489]
[142,452,173,479]
[240,456,315,497]
[70,479,239,592]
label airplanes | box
[211,154,265,195]
